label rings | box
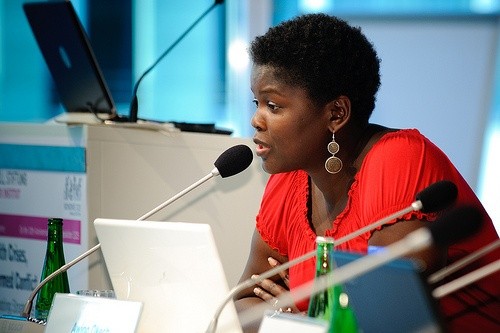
[273,299,280,307]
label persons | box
[230,13,500,333]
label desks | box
[83,126,270,297]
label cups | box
[77,290,115,299]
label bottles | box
[325,284,356,333]
[307,235,335,317]
[33,218,71,323]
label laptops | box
[94,218,244,333]
[23,0,215,133]
[333,251,446,333]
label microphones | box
[129,0,224,122]
[25,144,253,316]
[205,181,459,333]
[220,205,485,333]
